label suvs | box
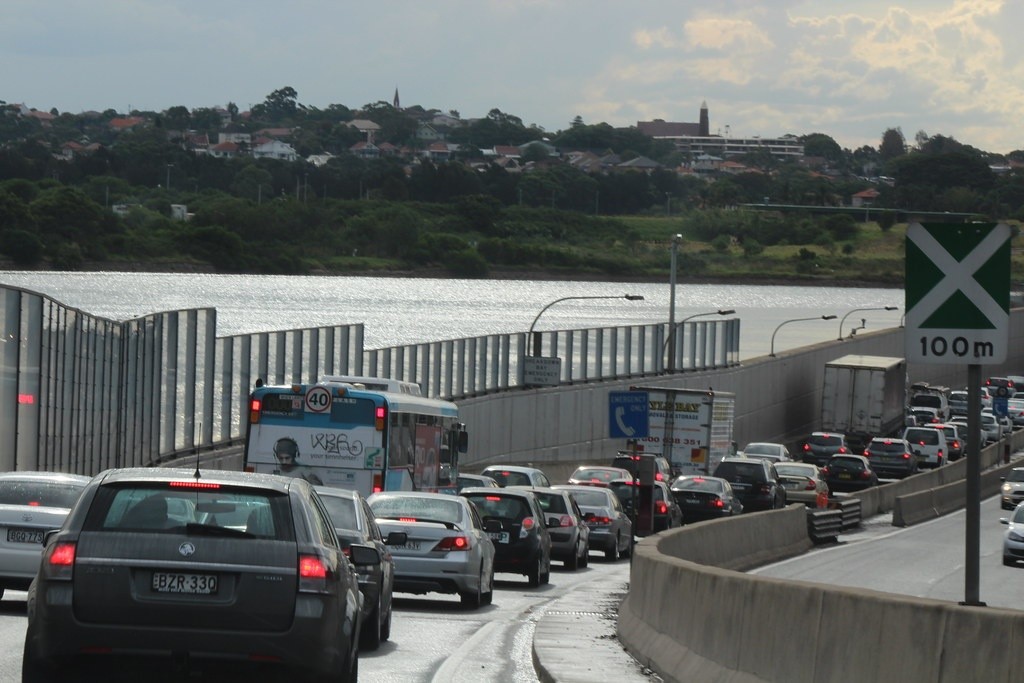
[900,375,1024,471]
[802,430,853,467]
[712,455,788,511]
[863,436,922,481]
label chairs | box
[246,505,274,536]
[122,495,163,527]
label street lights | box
[662,310,736,350]
[837,306,900,341]
[771,315,839,357]
[526,294,646,356]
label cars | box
[668,475,745,524]
[822,453,879,494]
[0,470,95,600]
[127,492,272,534]
[456,442,801,511]
[607,478,684,534]
[998,467,1024,510]
[310,485,408,652]
[546,484,634,562]
[1000,500,1024,567]
[363,490,504,611]
[22,467,382,682]
[457,487,562,589]
[501,485,597,573]
[770,461,830,510]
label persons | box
[276,438,323,486]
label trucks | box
[820,353,926,456]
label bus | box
[320,374,422,399]
[241,381,469,499]
